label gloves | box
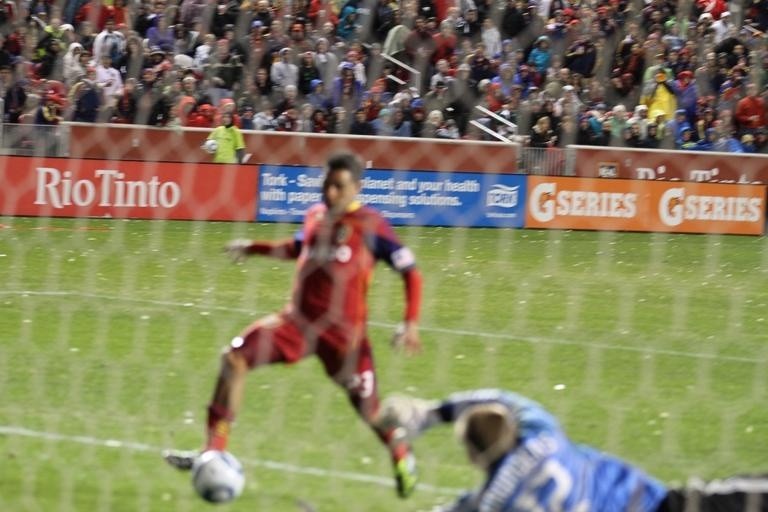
[369,393,441,447]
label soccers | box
[191,450,245,501]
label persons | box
[162,154,423,499]
[370,385,768,511]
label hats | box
[0,0,768,142]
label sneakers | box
[392,449,419,500]
[162,447,202,471]
